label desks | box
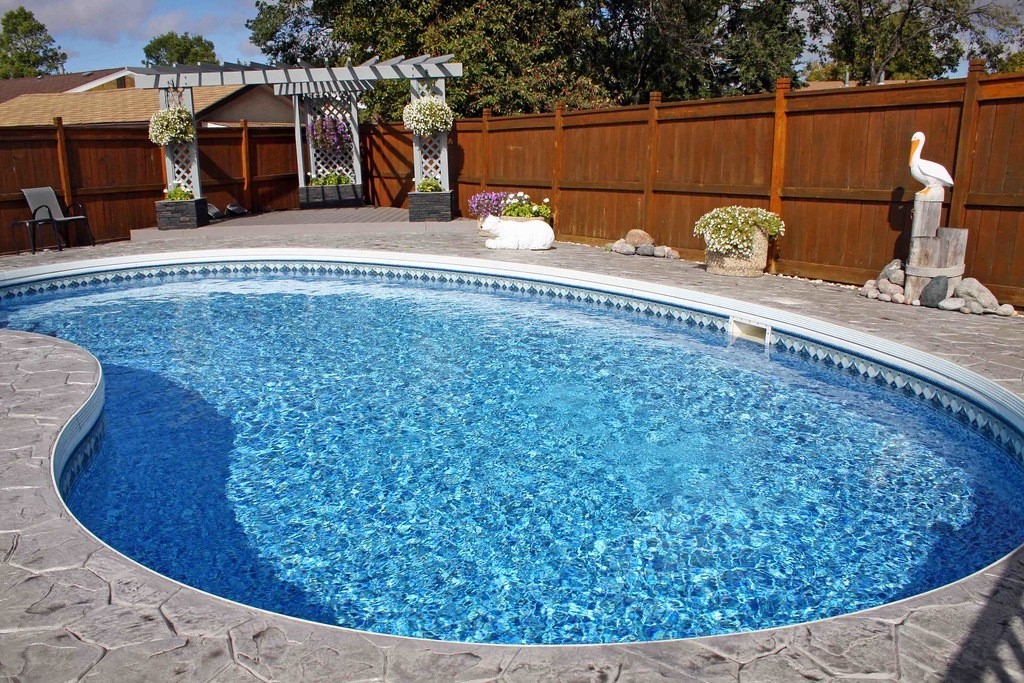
[11,219,61,256]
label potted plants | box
[407,177,455,223]
[299,173,363,208]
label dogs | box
[480,213,555,251]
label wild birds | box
[909,129,955,195]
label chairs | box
[20,186,95,251]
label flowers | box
[501,191,553,222]
[148,106,197,146]
[467,190,520,218]
[692,205,787,259]
[306,116,354,158]
[163,179,194,200]
[402,95,454,146]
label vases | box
[485,213,555,250]
[706,226,769,277]
[476,214,500,237]
[155,197,210,230]
